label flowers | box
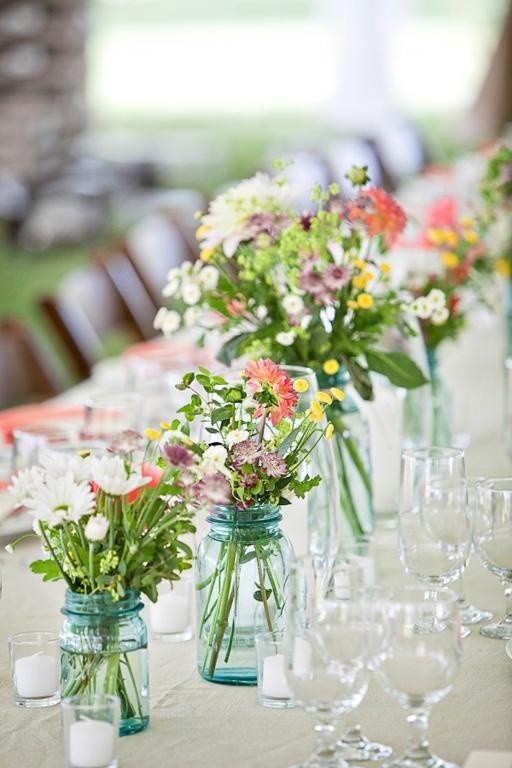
[153,145,511,538]
[6,420,198,720]
[170,358,345,678]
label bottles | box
[195,504,295,686]
[61,590,150,735]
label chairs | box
[0,110,427,409]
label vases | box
[195,505,295,687]
[308,363,375,555]
[58,588,149,738]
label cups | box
[9,630,62,708]
[63,696,122,768]
[255,630,299,711]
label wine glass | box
[283,448,512,768]
